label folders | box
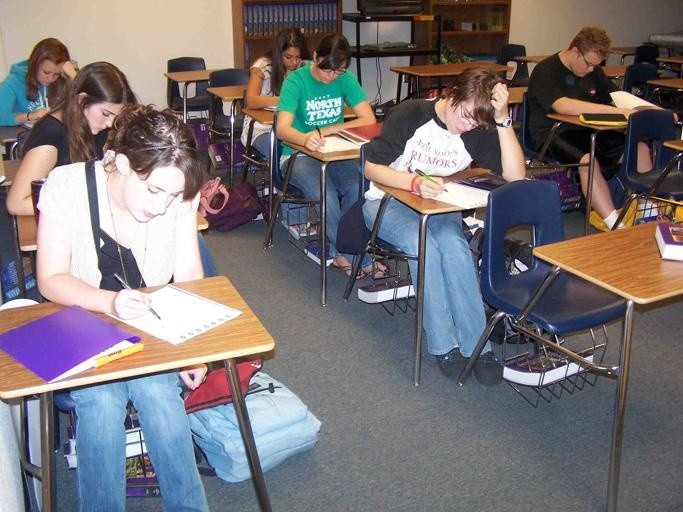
[243,3,339,37]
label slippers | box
[360,260,390,280]
[330,262,366,280]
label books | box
[340,121,382,142]
[280,216,316,241]
[426,168,511,212]
[578,110,630,127]
[652,219,683,263]
[204,139,250,170]
[499,342,597,389]
[242,4,338,36]
[354,279,415,304]
[301,238,335,267]
[335,130,366,145]
[52,412,167,498]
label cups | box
[30,178,51,232]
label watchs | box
[493,117,513,130]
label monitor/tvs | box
[356,0,423,16]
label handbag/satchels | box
[183,356,264,416]
[198,175,230,218]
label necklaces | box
[104,180,148,293]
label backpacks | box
[181,355,323,487]
[467,226,551,347]
[204,181,270,234]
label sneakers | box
[435,345,473,383]
[472,349,503,386]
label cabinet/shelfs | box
[412,0,511,90]
[232,0,342,115]
[342,13,441,87]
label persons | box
[0,37,80,133]
[237,26,319,241]
[271,32,390,286]
[360,64,530,385]
[31,106,214,512]
[4,59,140,219]
[527,25,659,230]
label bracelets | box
[26,111,30,121]
[408,173,418,193]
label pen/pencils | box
[45,96,49,109]
[91,343,145,368]
[414,168,449,193]
[315,124,322,139]
[112,273,160,321]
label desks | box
[0,158,210,297]
[0,125,28,158]
[0,275,277,512]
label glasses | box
[575,44,600,71]
[314,56,348,77]
[458,101,481,131]
